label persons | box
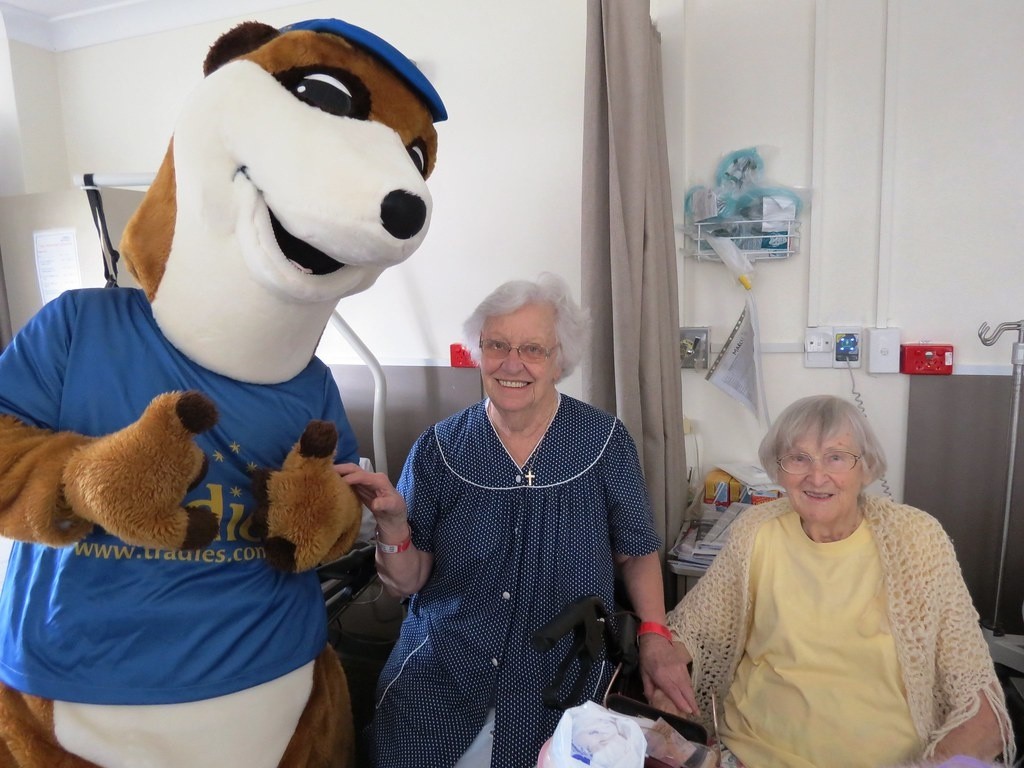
[335,278,703,768]
[651,392,1018,768]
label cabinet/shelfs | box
[0,185,147,341]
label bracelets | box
[374,524,413,554]
[634,620,675,648]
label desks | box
[317,552,379,628]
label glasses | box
[482,338,561,363]
[776,449,865,475]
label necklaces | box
[488,407,551,488]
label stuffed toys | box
[0,3,448,768]
